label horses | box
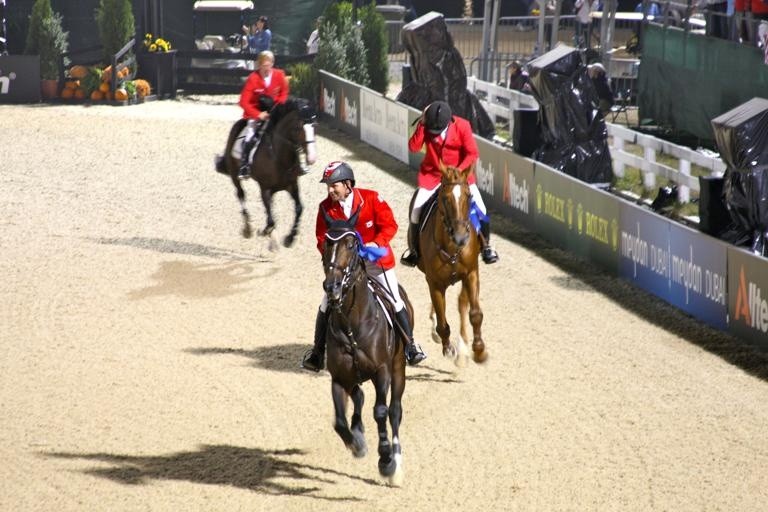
[318,203,415,489]
[406,157,491,364]
[214,95,318,248]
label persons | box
[307,15,324,53]
[506,61,528,90]
[636,1,659,16]
[689,0,768,45]
[237,51,316,178]
[402,101,498,266]
[240,15,272,70]
[587,62,614,118]
[574,1,601,50]
[303,162,427,364]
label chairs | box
[608,88,631,125]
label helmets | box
[320,161,355,188]
[425,101,452,135]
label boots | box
[478,220,497,264]
[395,306,424,365]
[401,222,420,267]
[237,139,252,178]
[303,305,328,370]
